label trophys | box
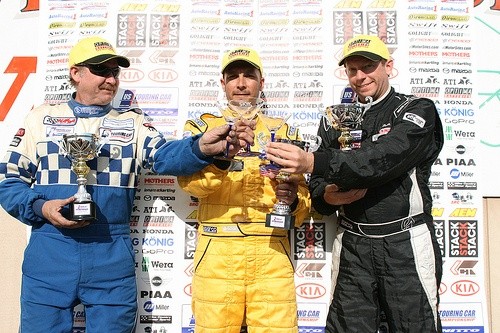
[256,132,323,230]
[317,95,373,150]
[52,131,110,222]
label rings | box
[348,201,351,204]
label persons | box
[265,34,444,333]
[178,48,311,333]
[0,38,236,333]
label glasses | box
[74,64,121,79]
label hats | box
[221,47,263,78]
[68,37,130,68]
[338,35,390,67]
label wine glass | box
[212,97,266,162]
